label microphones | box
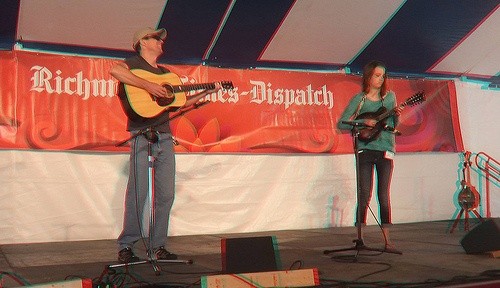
[384,123,400,136]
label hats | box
[132,27,167,48]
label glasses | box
[151,35,162,41]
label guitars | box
[458,151,481,211]
[118,69,233,123]
[354,92,426,141]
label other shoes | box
[384,244,402,254]
[154,247,178,260]
[118,247,140,263]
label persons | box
[337,61,404,249]
[108,26,222,262]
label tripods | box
[106,140,194,269]
[324,133,402,262]
[450,152,486,234]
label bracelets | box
[205,91,208,95]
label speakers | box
[461,219,500,253]
[221,235,283,273]
